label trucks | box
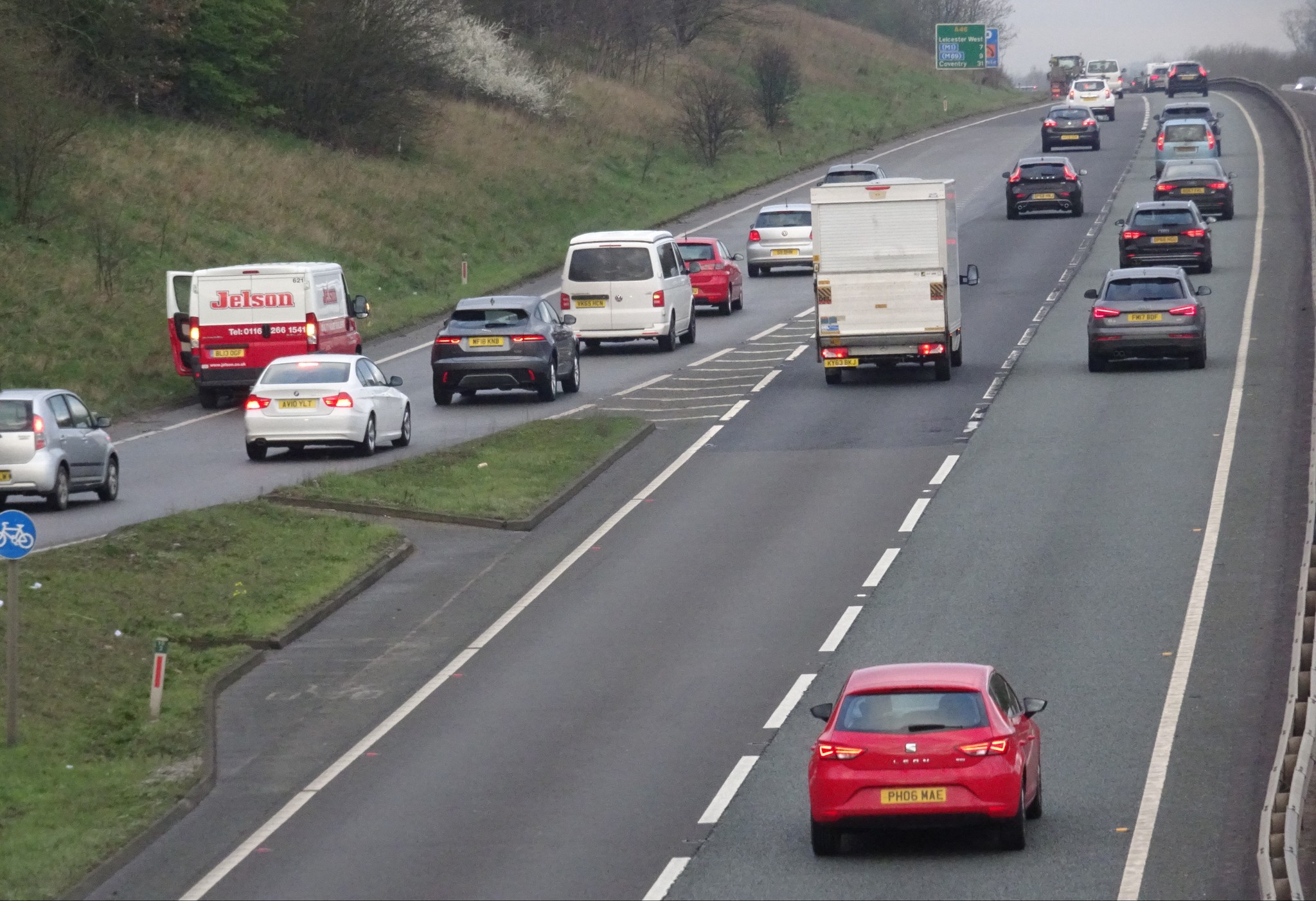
[809,177,962,386]
[1048,55,1082,100]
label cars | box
[1294,76,1316,92]
[808,661,1048,856]
[1002,156,1088,220]
[244,353,412,460]
[664,237,744,316]
[1129,61,1209,98]
[1039,104,1101,152]
[1151,118,1219,180]
[1065,76,1116,123]
[816,163,887,186]
[746,203,813,277]
[0,388,119,513]
[1149,158,1238,221]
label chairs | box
[852,697,902,732]
[940,695,978,725]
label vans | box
[1084,58,1127,100]
[166,259,369,410]
[559,230,696,351]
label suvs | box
[1153,101,1225,158]
[1114,199,1217,273]
[431,296,581,405]
[1083,266,1211,372]
